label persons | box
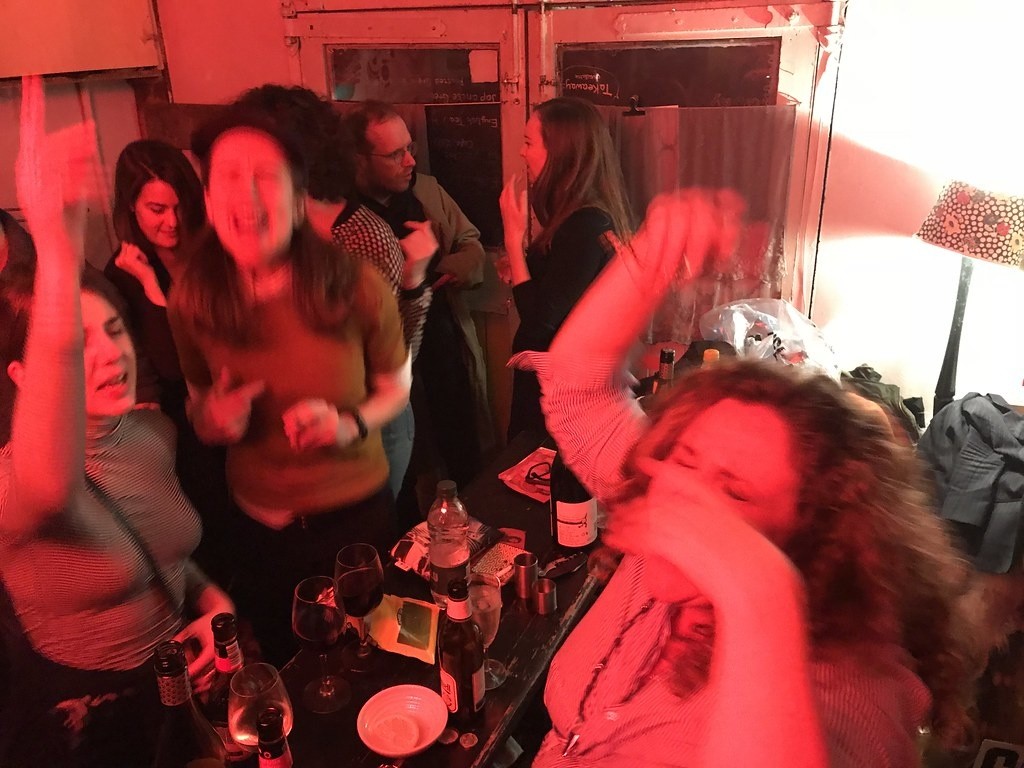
[506,186,986,768]
[194,85,415,499]
[345,98,488,489]
[497,95,637,443]
[170,115,412,662]
[0,76,236,768]
[102,140,208,417]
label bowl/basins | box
[357,684,448,756]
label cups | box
[228,663,292,752]
[532,577,558,615]
[513,552,538,599]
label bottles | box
[253,705,293,768]
[208,613,243,722]
[439,578,484,720]
[550,449,598,550]
[152,640,228,768]
[428,480,469,610]
[654,348,676,400]
[702,349,719,370]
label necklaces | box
[562,599,685,760]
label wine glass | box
[334,543,388,672]
[293,576,350,714]
[464,573,507,691]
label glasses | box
[353,141,417,164]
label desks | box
[65,429,625,768]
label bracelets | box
[605,229,646,284]
[349,408,369,438]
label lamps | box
[910,175,1024,418]
